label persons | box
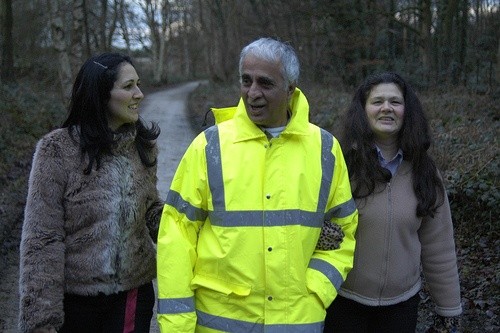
[314,73,462,333]
[18,52,165,333]
[157,36,358,333]
